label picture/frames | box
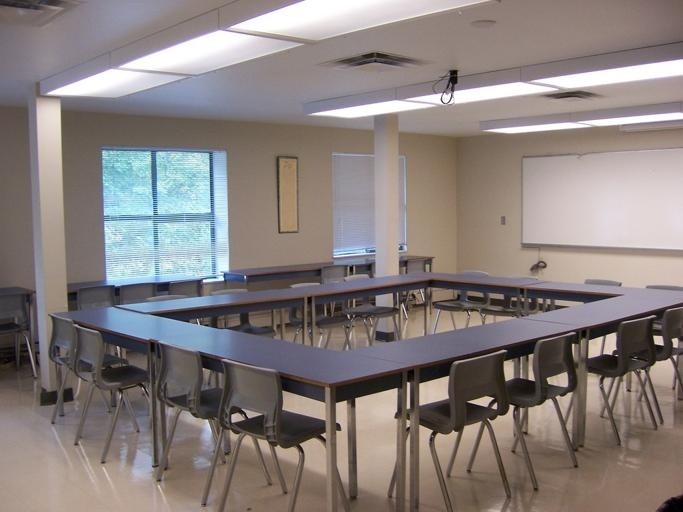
[275,155,299,233]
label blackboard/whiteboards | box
[520,146,683,253]
